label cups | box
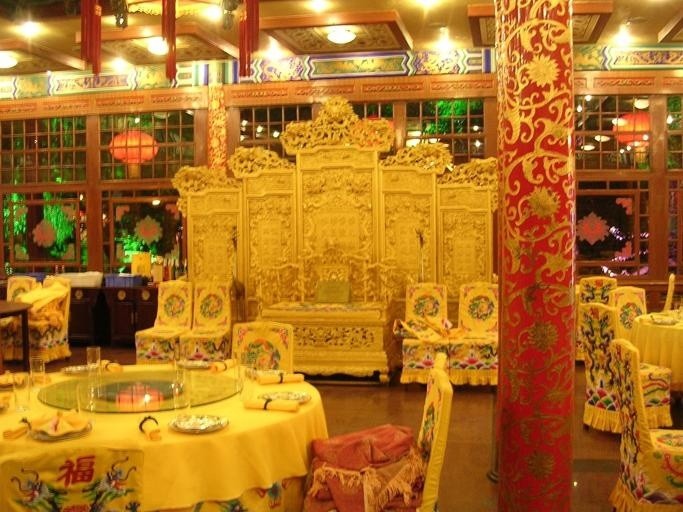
[171,368,190,413]
[85,345,102,372]
[28,354,47,394]
[11,372,31,416]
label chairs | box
[254,247,402,385]
[577,275,683,512]
[391,282,499,391]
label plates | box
[257,390,311,404]
[170,413,229,434]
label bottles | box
[74,383,95,417]
